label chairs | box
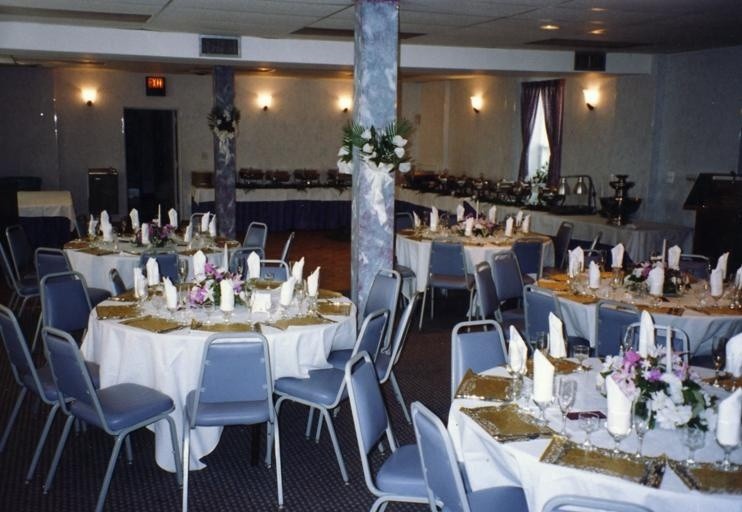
[343,349,445,511]
[410,399,526,512]
[265,309,390,484]
[1,304,101,485]
[328,292,418,427]
[543,494,646,511]
[41,326,182,511]
[450,320,509,402]
[1,212,741,363]
[182,331,285,511]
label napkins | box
[604,373,633,434]
[717,387,742,448]
[533,348,556,404]
[508,326,529,374]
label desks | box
[17,189,77,232]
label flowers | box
[336,116,417,175]
[208,105,241,141]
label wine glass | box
[89,229,217,256]
[565,263,741,310]
[423,213,530,244]
[501,343,742,473]
[134,256,320,326]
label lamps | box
[78,85,101,108]
[337,95,354,115]
[583,88,603,113]
[257,93,278,116]
[470,95,486,113]
